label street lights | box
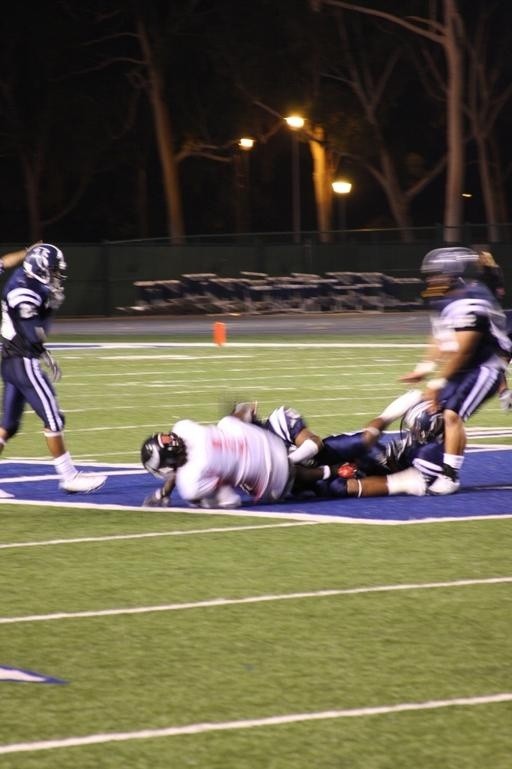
[284,110,307,242]
[330,177,353,242]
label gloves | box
[43,352,61,384]
[143,490,165,507]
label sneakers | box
[429,472,461,494]
[57,473,107,493]
[328,461,367,479]
[0,489,15,499]
[500,390,512,413]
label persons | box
[400,247,512,498]
[141,389,459,509]
[0,238,45,275]
[0,244,109,501]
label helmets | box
[141,433,187,478]
[420,248,479,299]
[399,402,443,444]
[23,244,67,292]
